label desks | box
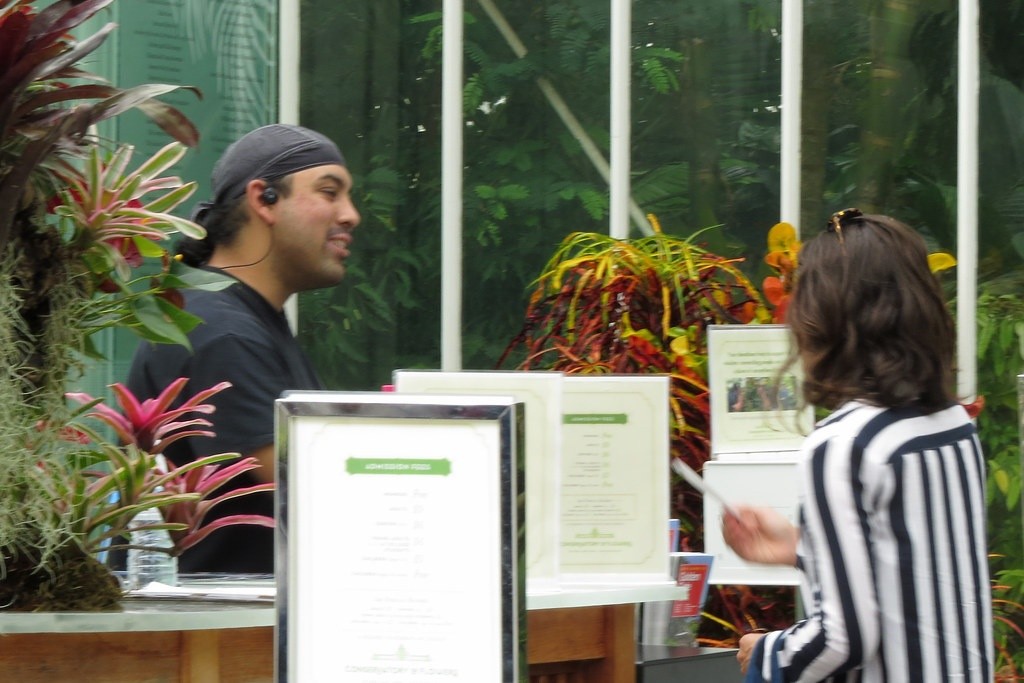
[0,560,692,683]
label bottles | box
[126,439,180,590]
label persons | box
[719,207,996,682]
[100,123,362,580]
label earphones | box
[264,187,277,204]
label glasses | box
[826,208,865,257]
